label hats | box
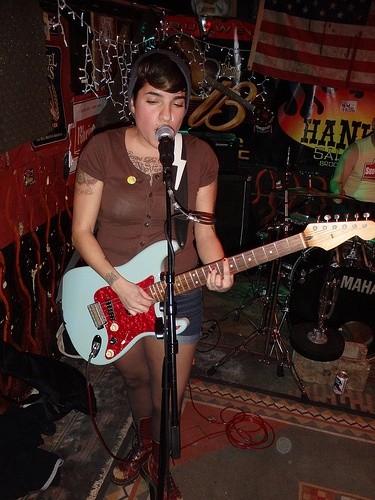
[127,49,191,116]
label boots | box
[141,439,183,500]
[110,413,153,485]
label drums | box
[287,239,375,357]
[263,213,323,270]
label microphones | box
[156,124,176,168]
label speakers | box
[215,175,256,256]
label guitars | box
[61,211,375,366]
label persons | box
[330,118,375,204]
[72,50,233,500]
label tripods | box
[208,147,310,403]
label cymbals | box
[288,186,353,199]
